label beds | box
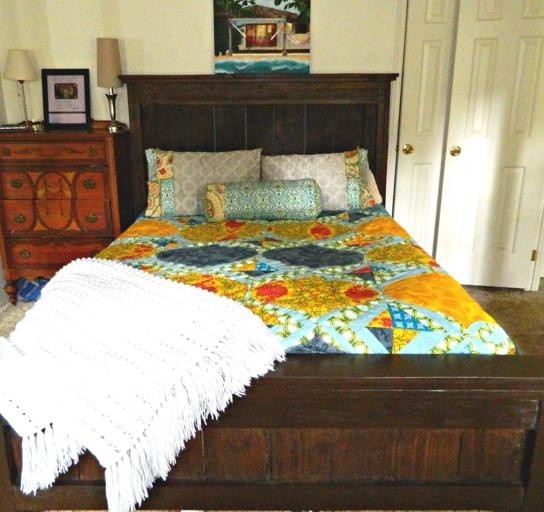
[0,73,544,511]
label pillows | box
[203,178,322,222]
[260,148,382,212]
[143,148,263,218]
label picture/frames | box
[41,69,90,127]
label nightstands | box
[0,120,134,307]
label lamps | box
[2,49,39,133]
[97,37,124,135]
